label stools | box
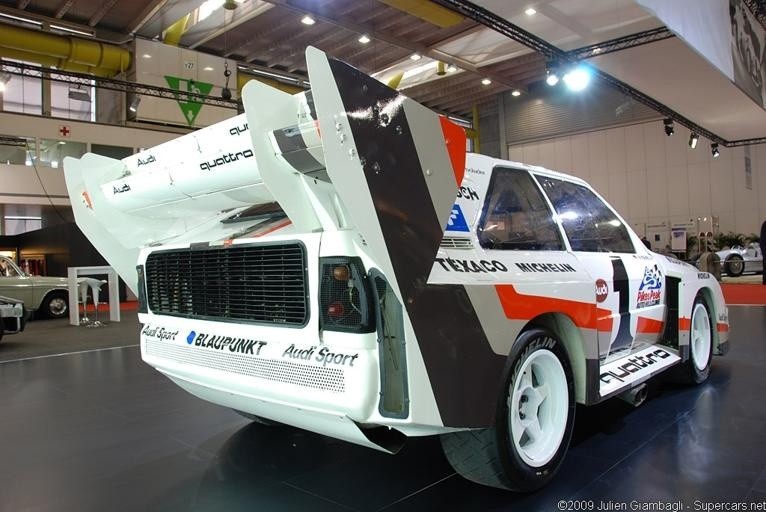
[76,277,92,325]
[82,278,108,328]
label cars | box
[0,255,69,341]
[696,242,763,277]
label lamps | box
[129,94,141,112]
[0,75,11,92]
[222,0,720,159]
[68,84,92,103]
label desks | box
[68,266,120,326]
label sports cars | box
[62,45,730,495]
